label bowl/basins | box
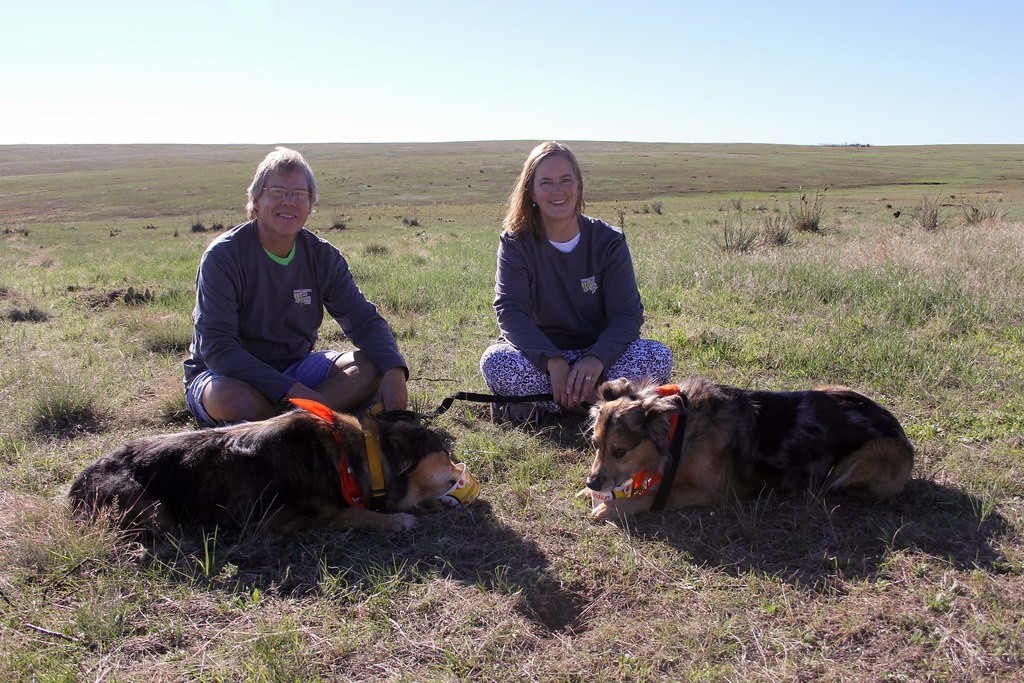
[437,462,481,508]
[586,477,633,513]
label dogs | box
[67,410,464,568]
[576,377,913,525]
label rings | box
[584,376,591,380]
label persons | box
[183,147,409,427]
[479,141,673,418]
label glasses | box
[262,187,313,202]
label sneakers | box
[490,398,551,425]
